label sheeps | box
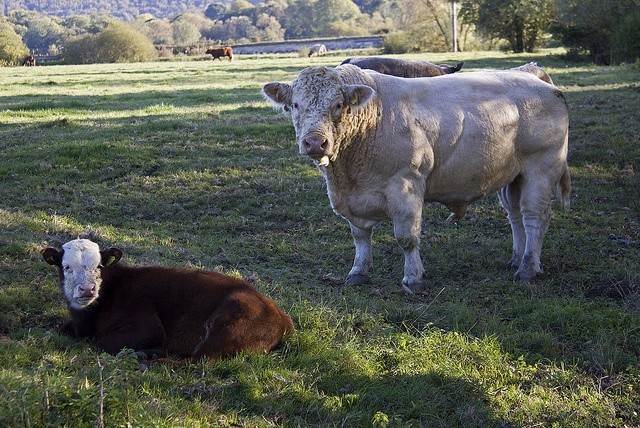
[40,230,294,367]
[341,58,464,78]
[21,55,35,66]
[205,47,233,62]
[262,62,572,295]
[308,44,327,57]
